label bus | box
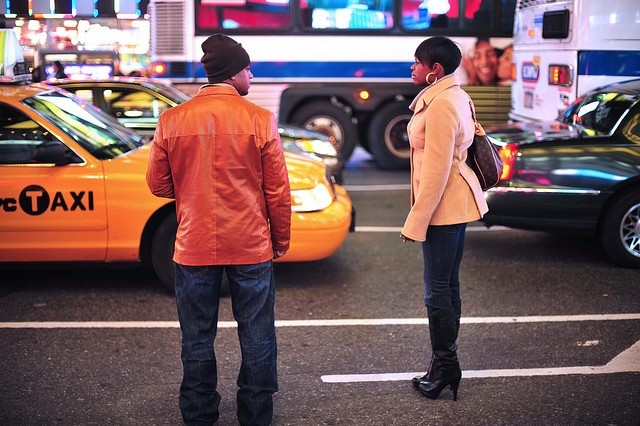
[508,0,639,126]
[149,0,517,169]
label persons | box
[53,60,76,95]
[462,37,497,86]
[145,33,292,425]
[31,66,42,83]
[397,35,504,401]
[498,44,512,85]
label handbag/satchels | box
[465,101,504,191]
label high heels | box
[418,351,461,401]
[412,353,457,390]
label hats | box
[201,33,251,84]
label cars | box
[478,79,639,267]
[0,72,354,297]
[37,78,344,185]
